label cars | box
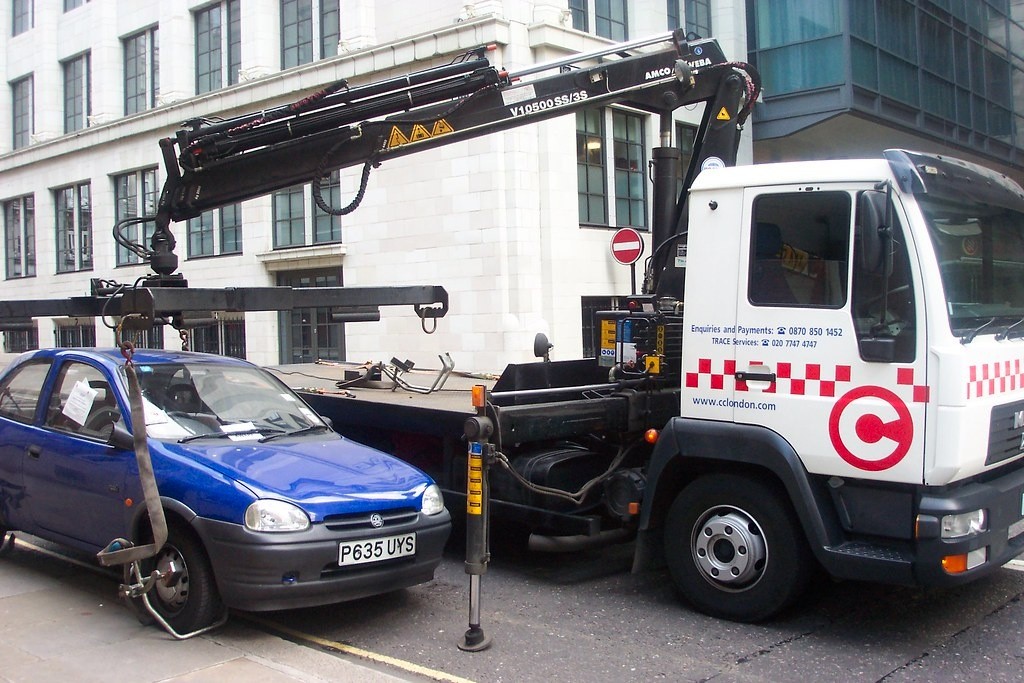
[0,346,453,633]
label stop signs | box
[610,227,644,265]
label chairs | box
[165,383,203,413]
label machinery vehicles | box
[0,29,1024,622]
